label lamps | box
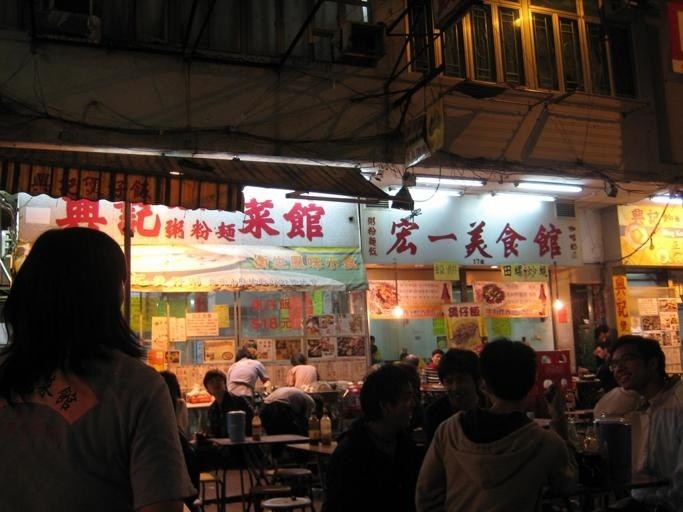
[606,187,618,197]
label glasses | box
[608,353,636,371]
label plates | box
[451,319,479,347]
[419,366,439,384]
[222,351,232,360]
[624,223,650,250]
[478,283,507,307]
[366,282,397,317]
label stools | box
[198,410,669,512]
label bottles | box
[251,405,262,441]
[440,283,450,304]
[320,403,332,447]
[308,405,318,446]
[564,388,575,411]
[566,416,578,438]
[539,285,546,315]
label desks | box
[203,434,311,510]
[419,383,445,403]
[287,441,338,504]
[572,376,600,408]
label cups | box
[226,410,244,442]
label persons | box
[0,228,198,511]
[429,349,443,369]
[594,323,620,393]
[607,335,683,511]
[257,387,316,435]
[226,339,271,407]
[287,353,321,390]
[160,371,189,437]
[393,353,418,367]
[200,369,255,439]
[422,349,488,443]
[322,365,420,511]
[370,335,377,365]
[415,338,567,512]
